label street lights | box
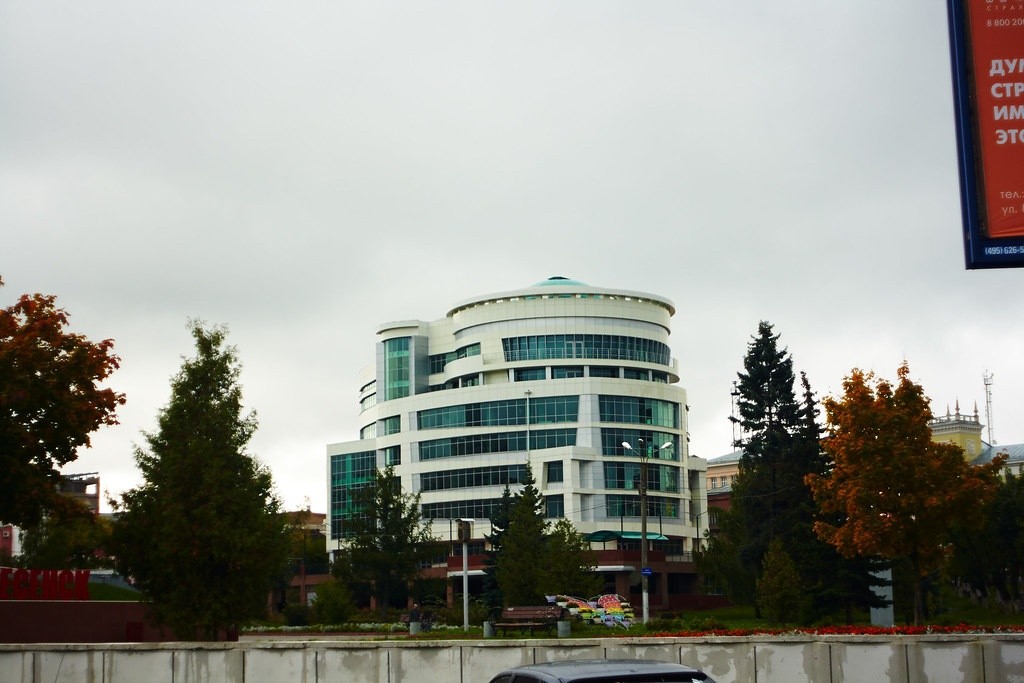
[619,436,671,625]
[436,513,465,556]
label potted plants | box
[544,591,634,625]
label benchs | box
[398,611,435,631]
[486,602,568,639]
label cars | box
[487,657,716,683]
